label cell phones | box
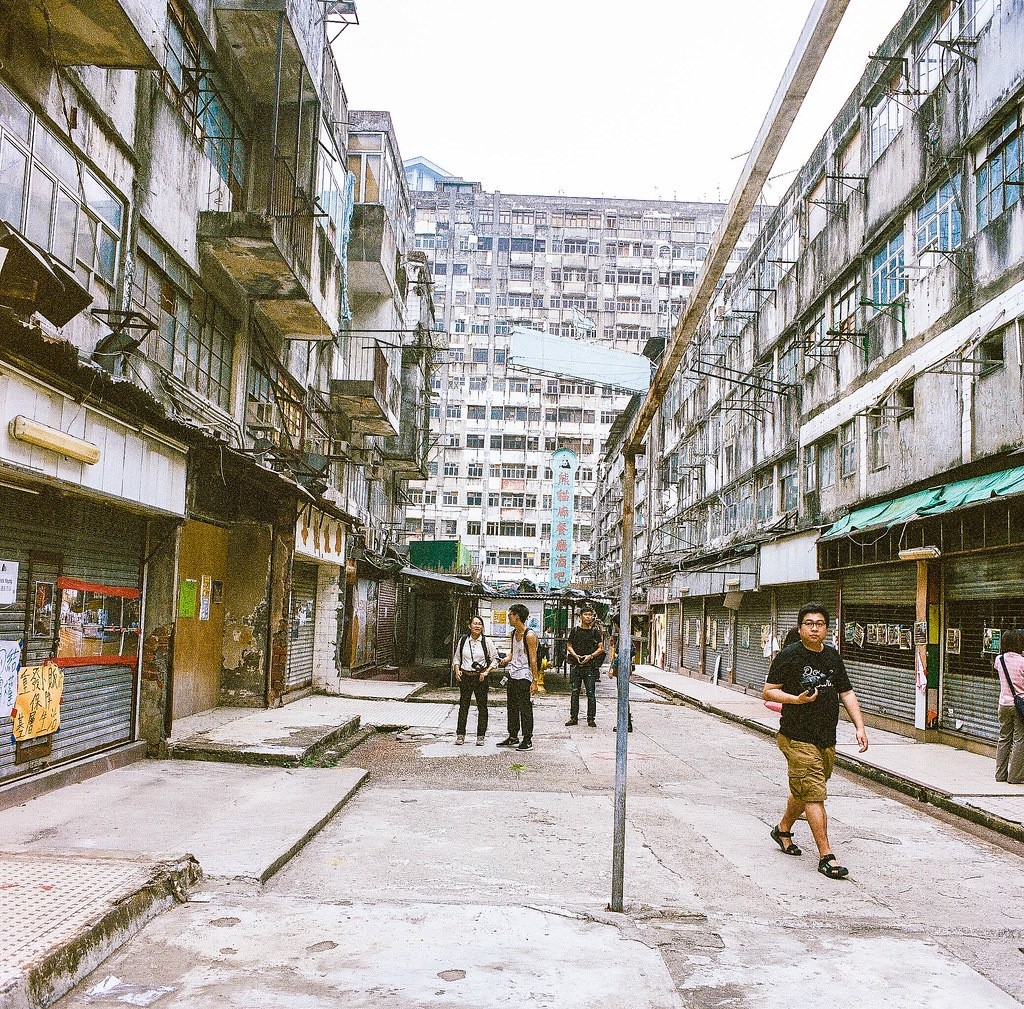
[579,656,585,662]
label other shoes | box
[588,720,596,727]
[614,725,632,732]
[565,720,577,726]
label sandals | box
[818,854,848,878]
[771,826,802,855]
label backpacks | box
[512,628,541,670]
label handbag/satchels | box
[1013,694,1024,723]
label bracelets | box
[532,679,538,683]
[590,654,595,659]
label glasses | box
[801,620,826,628]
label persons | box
[564,606,604,728]
[992,628,1024,784]
[541,624,552,663]
[608,613,635,732]
[451,615,501,746]
[779,625,813,820]
[495,603,540,751]
[760,602,869,878]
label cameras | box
[472,662,484,673]
[802,674,827,697]
[499,676,508,686]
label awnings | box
[0,302,317,505]
[815,464,1023,543]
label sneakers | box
[455,735,464,744]
[476,736,483,745]
[516,741,533,751]
[496,738,519,747]
